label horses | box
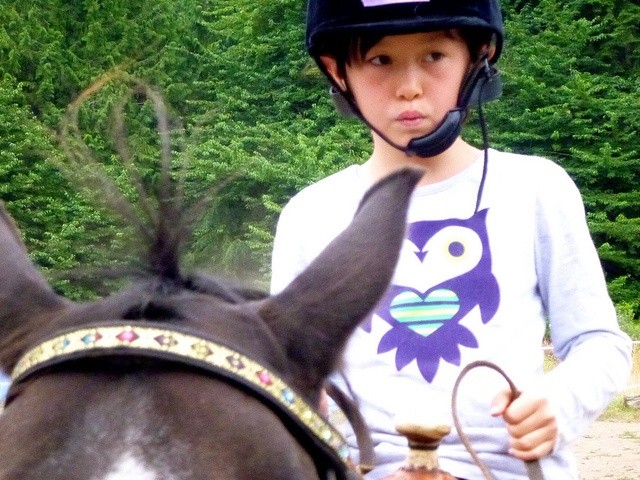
[0,168,426,480]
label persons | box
[269,0,635,479]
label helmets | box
[305,0,501,158]
